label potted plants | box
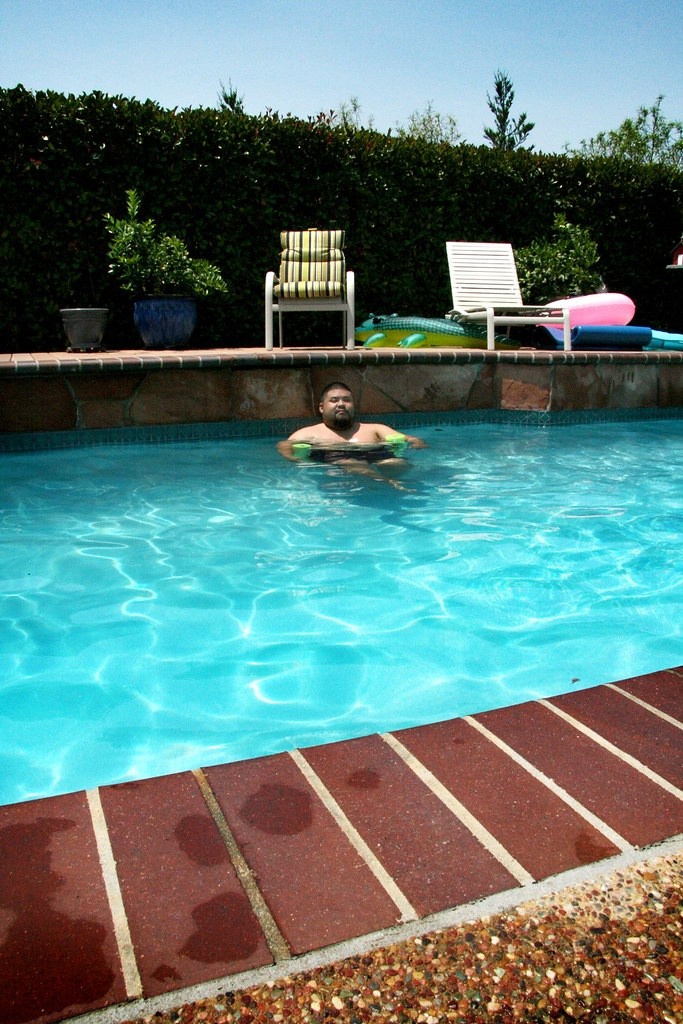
[103,188,229,349]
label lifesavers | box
[537,292,636,329]
[291,433,407,465]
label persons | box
[276,382,427,492]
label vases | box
[60,307,107,350]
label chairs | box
[264,228,355,350]
[446,241,571,351]
